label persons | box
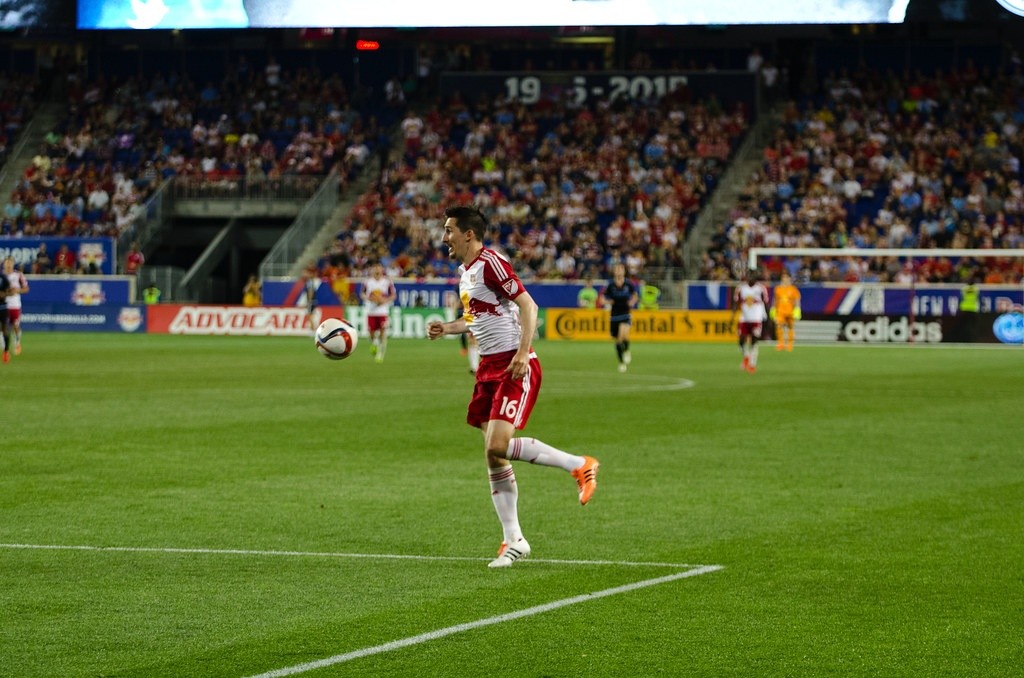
[1,48,1023,372]
[427,205,601,569]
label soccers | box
[315,317,358,361]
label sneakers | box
[571,456,599,506]
[488,535,532,569]
